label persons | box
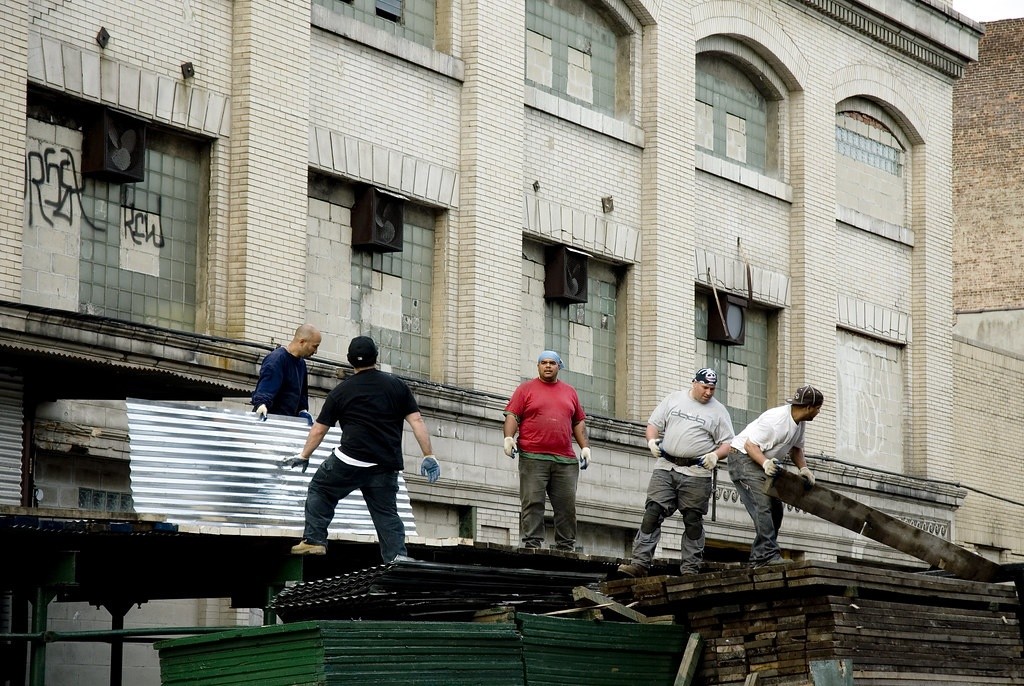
[502,350,593,551]
[281,335,441,563]
[250,325,321,425]
[727,384,824,568]
[616,368,735,578]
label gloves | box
[798,467,815,486]
[256,404,268,420]
[698,452,718,471]
[421,455,441,483]
[283,454,310,474]
[648,438,663,458]
[762,458,781,476]
[504,437,517,459]
[299,409,314,424]
[580,447,591,470]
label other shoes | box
[618,563,648,578]
[762,557,793,566]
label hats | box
[346,336,379,367]
[785,384,823,405]
[692,370,717,385]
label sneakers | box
[290,540,326,555]
